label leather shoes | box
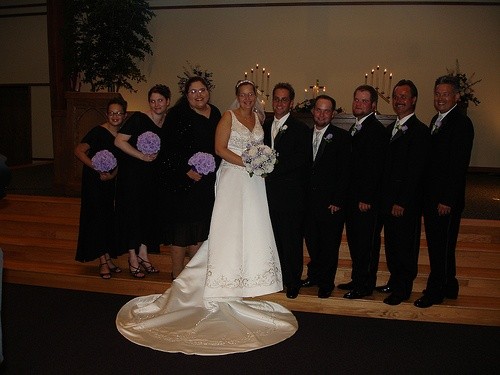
[343,290,372,299]
[318,284,336,298]
[336,281,352,291]
[300,278,315,288]
[383,292,409,305]
[421,288,458,300]
[376,284,393,293]
[285,283,302,299]
[414,290,443,309]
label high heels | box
[137,256,160,274]
[128,259,145,279]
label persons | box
[414,75,474,308]
[114,84,172,278]
[376,78,428,305]
[258,83,301,299]
[75,97,128,281]
[169,76,222,281]
[336,84,389,299]
[298,95,350,299]
[212,78,298,298]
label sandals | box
[106,258,121,274]
[99,262,113,279]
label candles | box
[381,69,387,92]
[251,68,254,81]
[365,73,368,85]
[266,71,270,96]
[261,67,265,91]
[244,71,248,78]
[375,65,379,89]
[255,64,259,87]
[387,71,393,98]
[369,69,374,86]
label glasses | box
[272,97,289,104]
[108,111,125,116]
[188,89,207,96]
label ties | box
[272,121,280,140]
[392,121,400,138]
[434,115,443,125]
[313,131,321,161]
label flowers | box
[433,121,441,129]
[280,125,287,133]
[354,122,363,132]
[444,68,483,104]
[137,131,161,155]
[321,134,334,142]
[188,153,215,175]
[241,142,280,177]
[91,151,117,172]
[397,125,408,132]
